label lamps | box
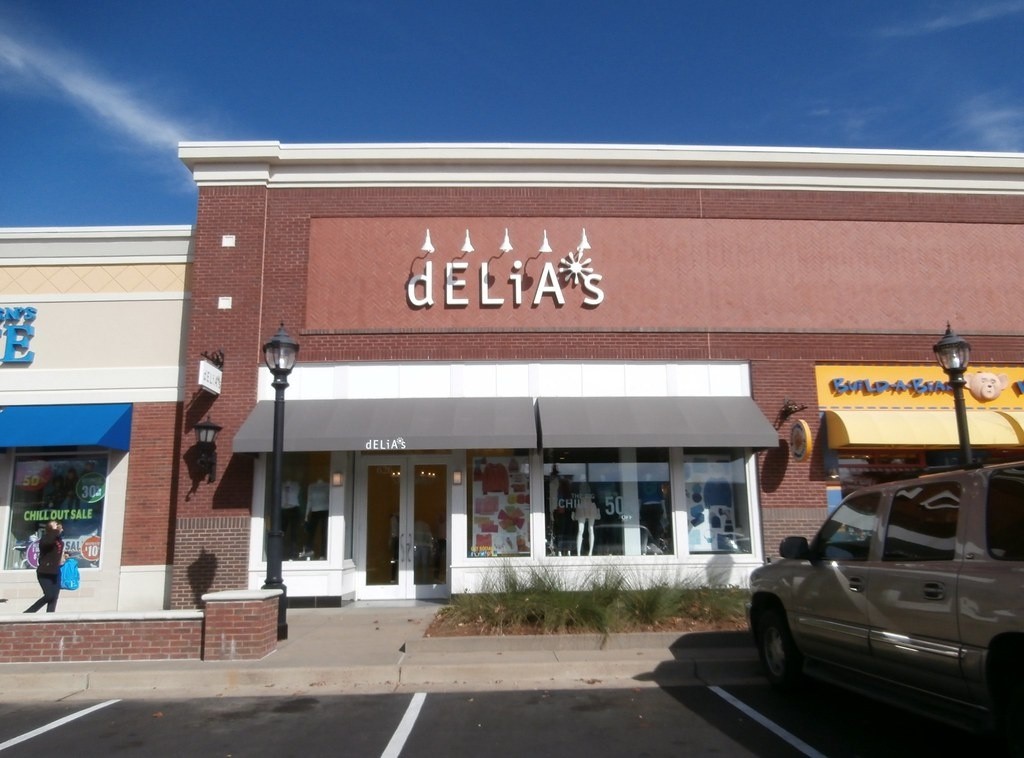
[499,228,513,253]
[421,229,434,253]
[578,227,592,249]
[193,415,223,483]
[539,230,553,252]
[461,229,474,252]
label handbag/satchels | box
[59,558,80,590]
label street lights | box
[931,319,979,468]
[260,320,300,640]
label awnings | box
[231,398,781,453]
[827,409,1023,449]
[0,404,132,453]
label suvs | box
[591,523,664,556]
[744,459,1024,740]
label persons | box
[544,465,601,556]
[279,481,302,561]
[389,512,445,584]
[305,478,329,560]
[23,521,64,612]
[49,461,94,520]
